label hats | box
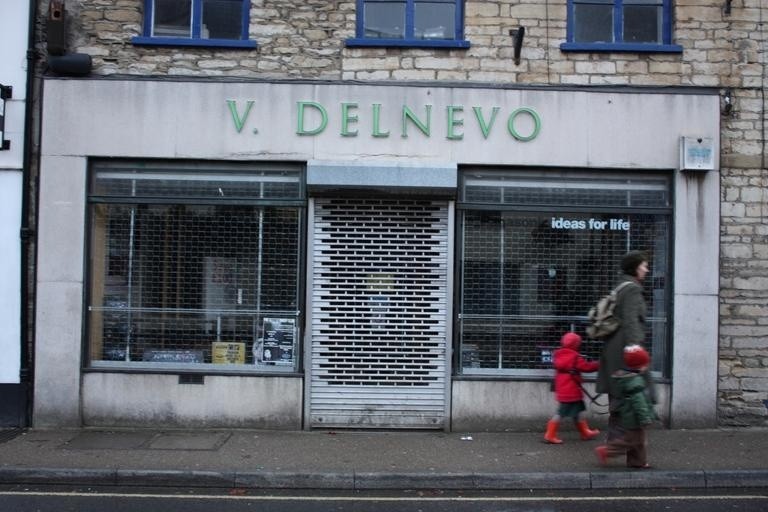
[623,344,651,370]
[620,250,647,278]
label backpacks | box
[585,281,634,341]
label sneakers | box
[594,446,608,466]
[642,462,651,470]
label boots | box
[542,419,563,444]
[575,418,601,441]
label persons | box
[541,333,602,445]
[595,249,660,452]
[592,341,657,468]
